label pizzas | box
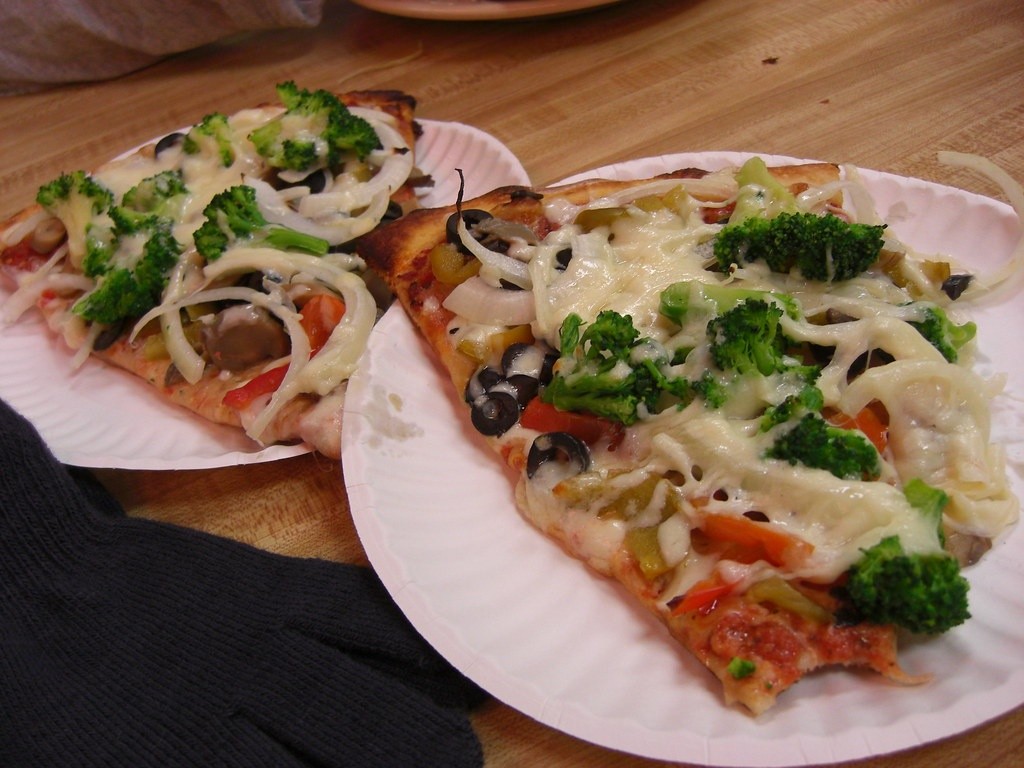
[355,153,1024,713]
[0,75,436,463]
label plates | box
[340,152,1024,768]
[0,106,534,472]
[355,0,616,21]
